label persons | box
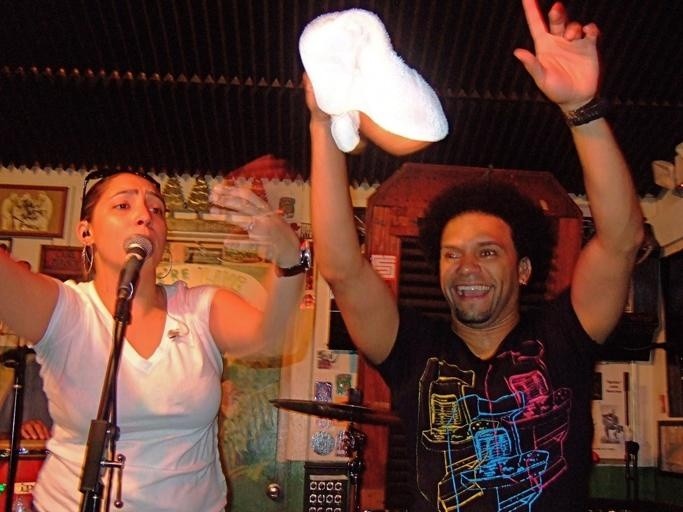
[0,358,53,440]
[0,189,54,233]
[0,169,307,512]
[299,1,646,512]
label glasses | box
[81,167,162,206]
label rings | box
[240,216,255,233]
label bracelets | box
[561,99,610,129]
[274,247,313,277]
[564,95,602,121]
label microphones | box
[0,341,35,362]
[114,234,153,321]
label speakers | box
[582,217,659,362]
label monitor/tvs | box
[328,208,367,354]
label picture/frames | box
[39,244,85,283]
[658,421,683,478]
[0,183,68,238]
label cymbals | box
[267,399,400,425]
